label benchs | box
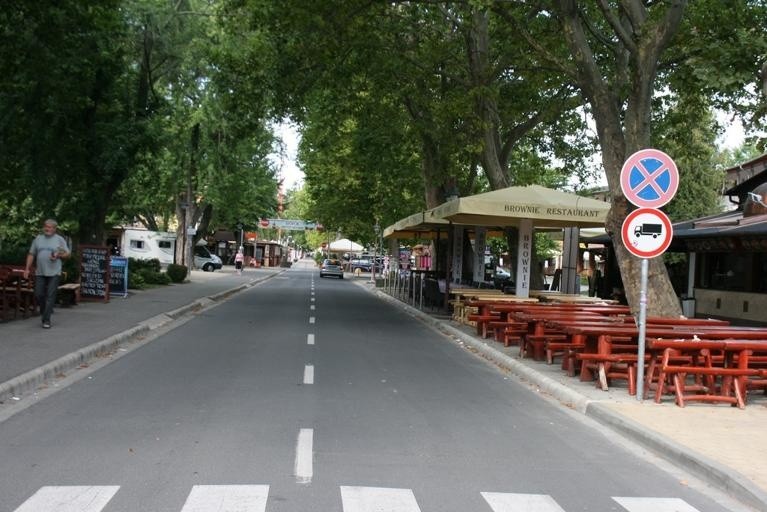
[449,289,767,409]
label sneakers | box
[41,319,51,329]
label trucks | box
[120,230,222,271]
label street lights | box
[373,222,382,278]
[237,223,244,272]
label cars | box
[496,266,510,281]
[320,259,343,279]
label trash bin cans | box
[682,298,696,318]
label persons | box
[24,219,73,329]
[233,249,244,276]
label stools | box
[57,282,81,308]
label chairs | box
[408,269,523,312]
[0,263,37,323]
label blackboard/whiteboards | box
[75,244,128,296]
